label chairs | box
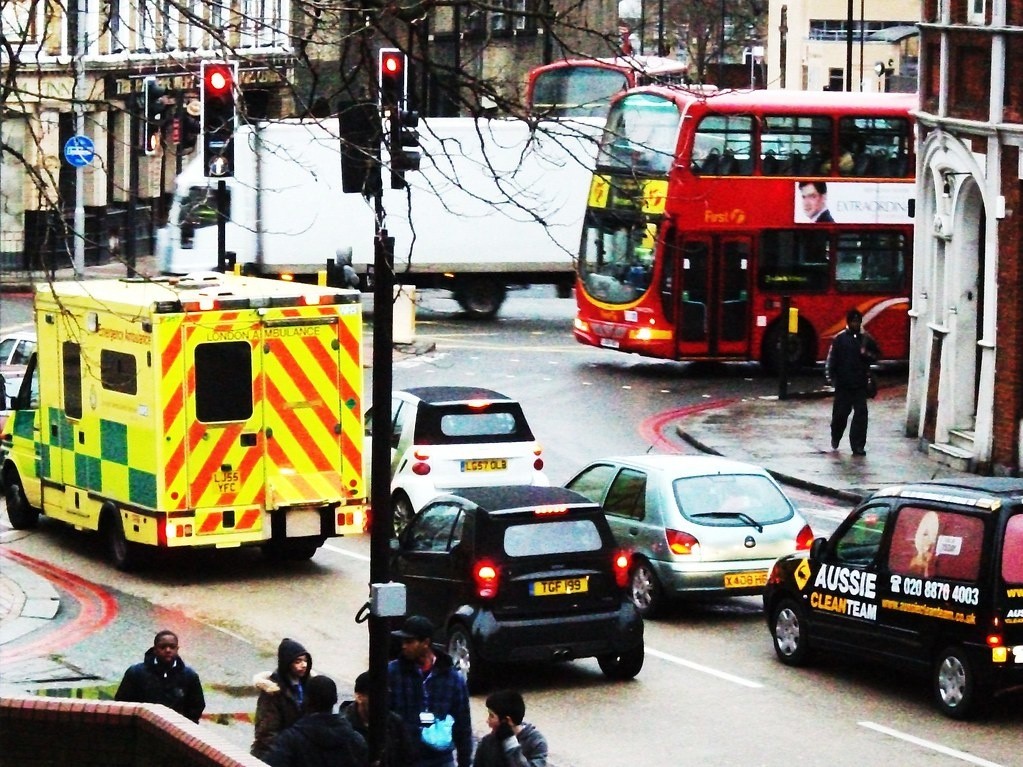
[698,144,917,178]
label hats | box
[390,615,434,640]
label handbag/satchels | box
[866,373,877,399]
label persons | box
[251,615,417,767]
[472,691,548,767]
[115,631,207,725]
[816,308,881,457]
[386,616,474,767]
[798,182,835,222]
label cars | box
[761,476,1023,720]
[560,455,815,621]
[364,386,550,539]
[0,331,38,402]
[387,485,646,697]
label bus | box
[526,56,690,118]
[573,81,920,379]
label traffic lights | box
[391,110,420,171]
[378,48,408,111]
[200,60,239,149]
[144,76,169,133]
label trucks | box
[152,118,606,321]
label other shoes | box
[832,435,839,449]
[853,447,867,457]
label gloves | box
[494,723,514,741]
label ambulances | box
[0,271,367,571]
